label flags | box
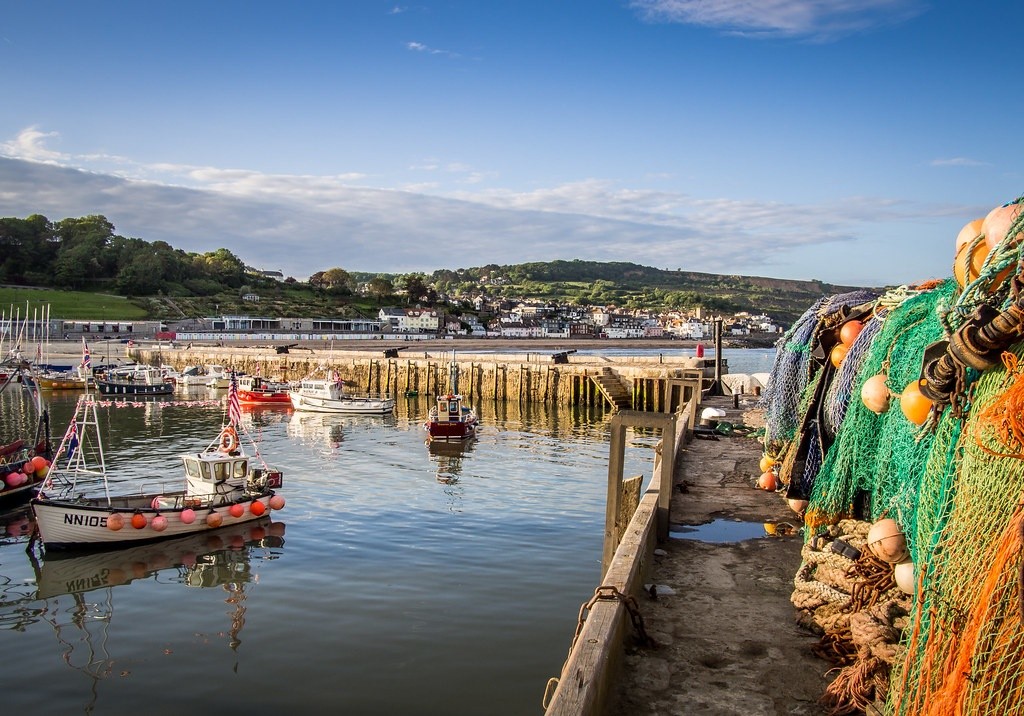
[83,342,91,369]
[67,426,78,460]
[334,370,342,391]
[228,371,240,425]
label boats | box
[0,299,238,390]
[25,516,289,712]
[93,377,174,396]
[0,355,65,522]
[288,411,395,449]
[427,395,478,441]
[287,338,400,414]
[426,440,477,494]
[239,373,297,406]
[26,373,286,548]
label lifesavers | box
[220,427,239,453]
